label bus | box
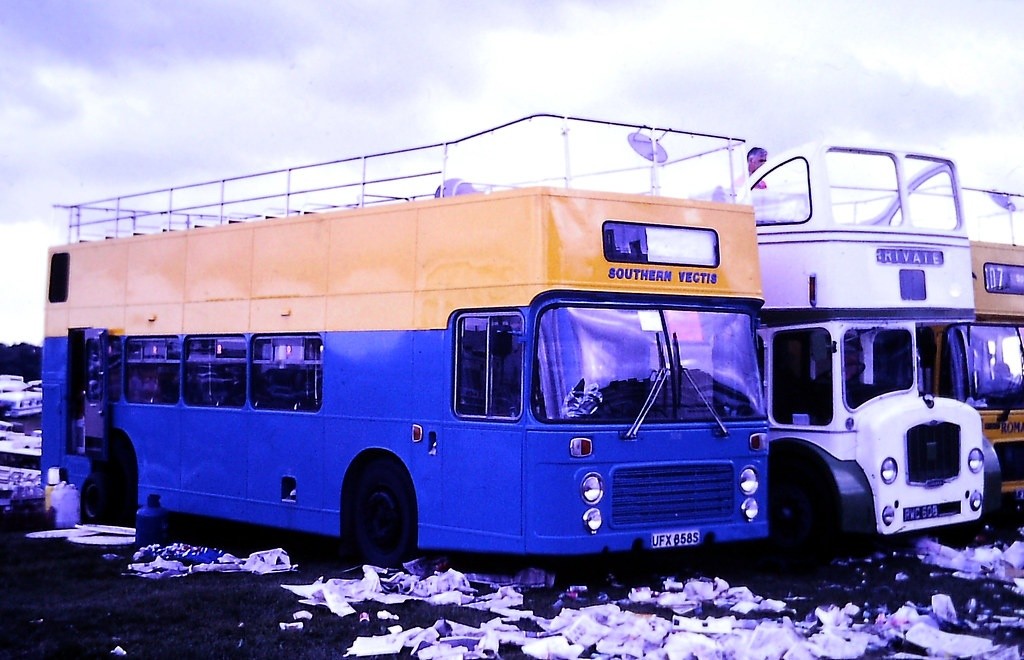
[42,113,770,568]
[695,147,1024,571]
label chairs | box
[993,362,1011,379]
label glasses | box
[760,159,766,162]
